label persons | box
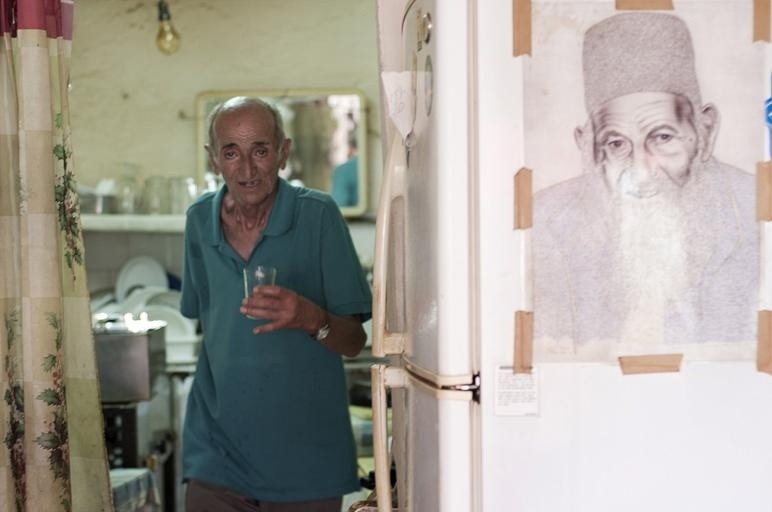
[532,12,756,359]
[180,95,373,510]
[331,130,359,208]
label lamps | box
[155,0,181,55]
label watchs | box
[312,312,332,343]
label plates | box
[84,255,202,333]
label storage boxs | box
[349,405,393,457]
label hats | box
[580,9,703,115]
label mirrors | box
[195,87,369,219]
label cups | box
[239,266,280,321]
[114,173,220,216]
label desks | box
[110,467,161,512]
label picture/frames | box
[510,0,772,376]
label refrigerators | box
[372,1,480,512]
